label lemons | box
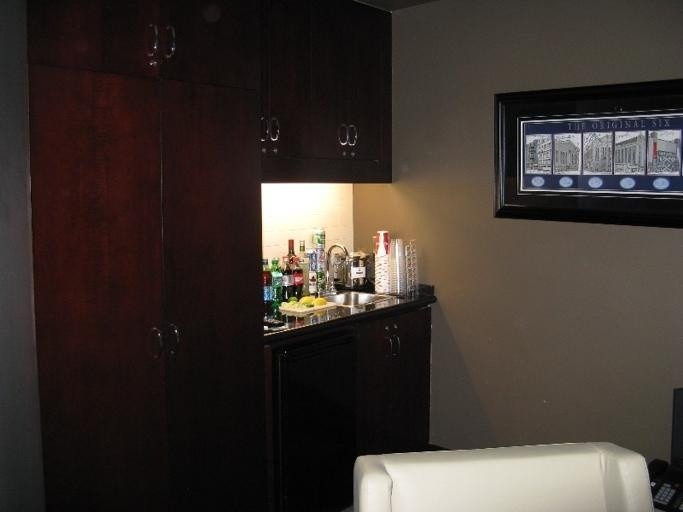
[280,296,328,312]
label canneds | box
[309,271,317,295]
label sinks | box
[323,291,396,308]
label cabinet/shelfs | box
[25,0,437,512]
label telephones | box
[646,459,683,512]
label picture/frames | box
[493,78,683,229]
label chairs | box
[350,441,655,511]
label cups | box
[372,230,419,296]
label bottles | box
[262,238,309,320]
[331,252,345,283]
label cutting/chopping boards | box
[279,302,336,316]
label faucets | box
[326,243,348,281]
